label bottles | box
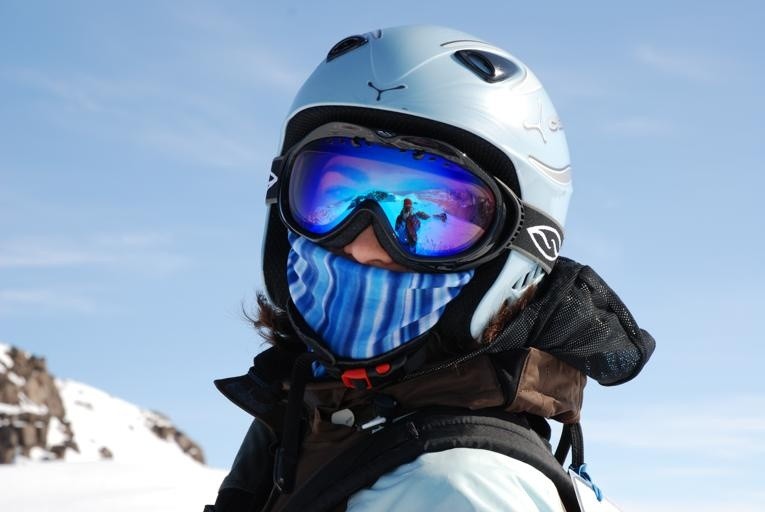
[404,199,411,207]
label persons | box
[394,197,423,257]
[203,24,656,511]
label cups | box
[276,122,527,273]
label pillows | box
[260,26,570,344]
